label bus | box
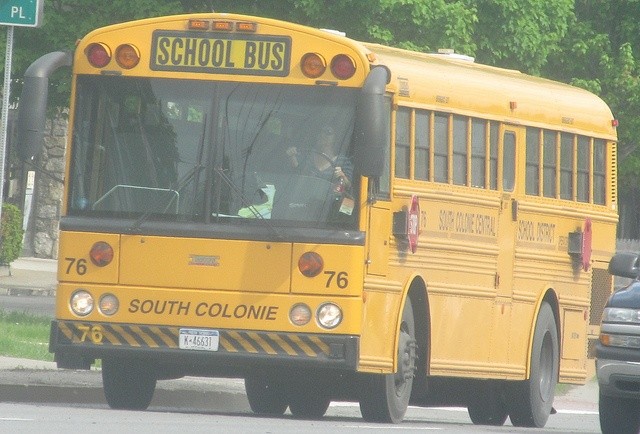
[48,12,618,429]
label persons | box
[285,117,354,191]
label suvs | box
[595,243,637,434]
